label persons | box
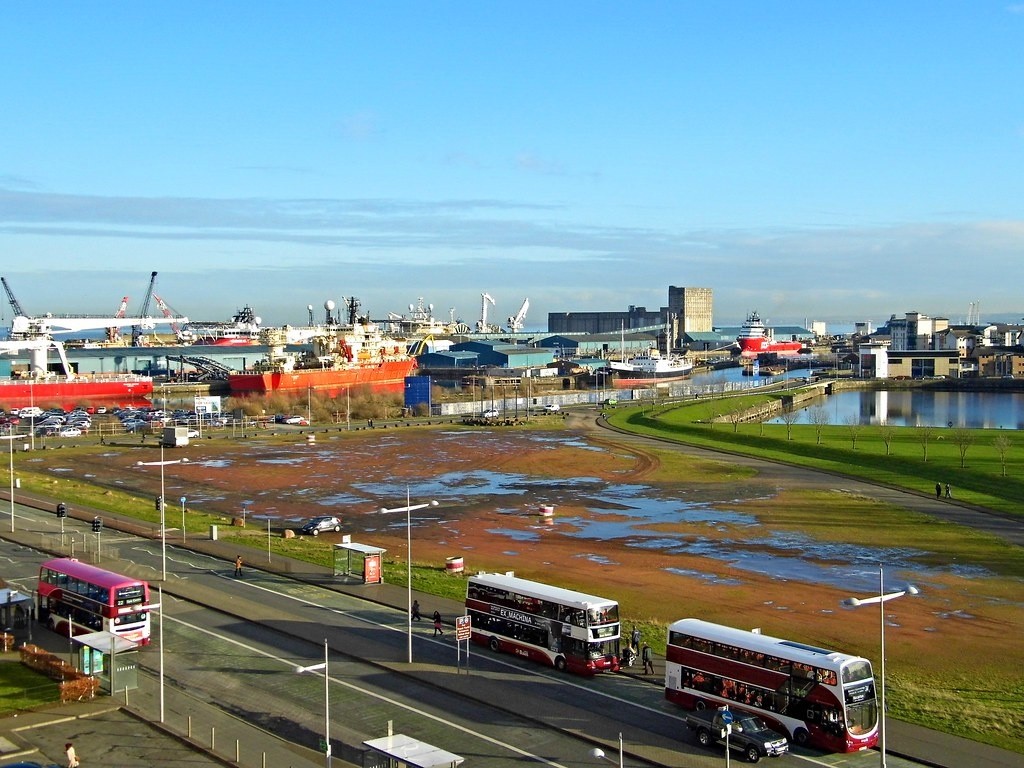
[936,483,941,499]
[411,600,422,621]
[945,483,952,499]
[681,638,853,705]
[235,556,243,576]
[432,611,443,637]
[625,625,656,674]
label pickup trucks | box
[685,708,789,764]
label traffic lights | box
[58,505,103,532]
[155,497,160,510]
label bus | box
[664,617,879,753]
[465,573,621,677]
[37,558,151,649]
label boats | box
[0,297,456,400]
[736,308,802,360]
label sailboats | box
[606,311,693,380]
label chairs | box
[684,637,835,707]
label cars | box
[301,515,342,535]
[482,399,618,418]
[0,405,309,438]
[894,374,1014,380]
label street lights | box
[588,732,623,768]
[377,488,439,664]
[292,638,331,768]
[0,425,34,532]
[136,445,190,582]
[844,567,919,768]
[131,585,164,724]
